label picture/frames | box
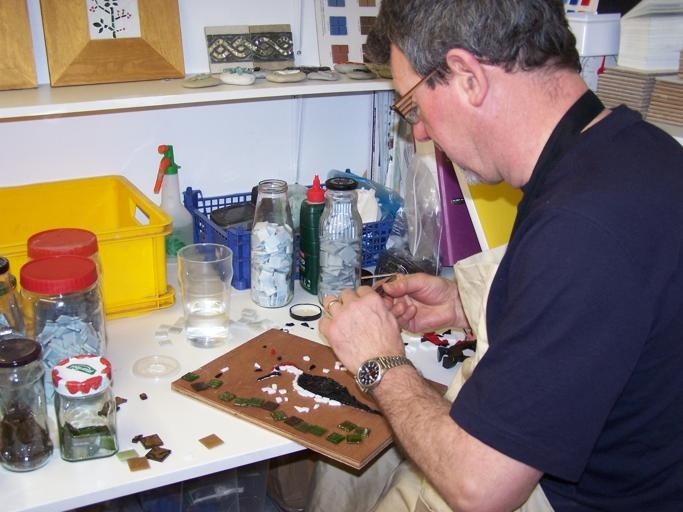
[37,1,187,88]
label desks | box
[0,252,483,512]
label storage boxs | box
[566,11,622,58]
[0,172,177,335]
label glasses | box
[390,68,439,125]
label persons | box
[319,0,682,508]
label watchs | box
[355,354,415,401]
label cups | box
[176,242,235,347]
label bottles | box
[52,354,119,462]
[20,256,106,404]
[1,339,52,472]
[319,176,363,306]
[300,173,327,293]
[0,257,25,332]
[27,226,98,257]
[250,179,294,309]
